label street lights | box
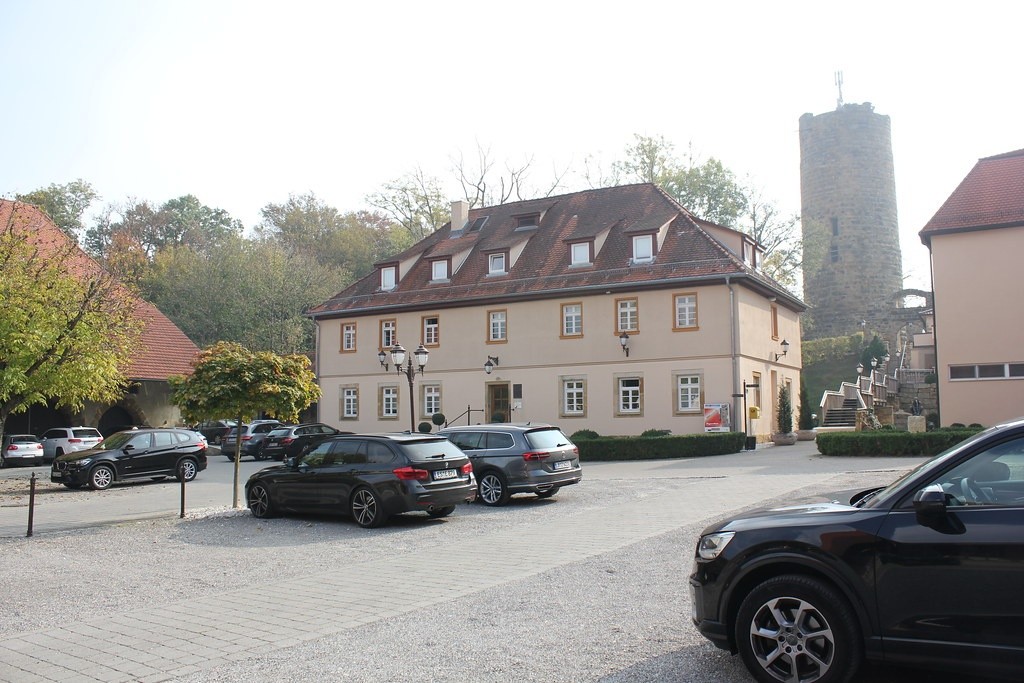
[377,341,430,433]
[884,350,890,376]
[870,355,878,384]
[895,349,901,369]
[856,363,864,393]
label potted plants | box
[771,384,798,446]
[793,376,817,441]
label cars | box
[1,434,45,467]
[245,431,477,528]
[688,414,1024,683]
[189,419,238,445]
[262,423,357,461]
[39,425,105,463]
[434,420,582,507]
[50,428,207,490]
[221,420,287,461]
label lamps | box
[484,355,498,374]
[378,349,389,372]
[775,340,790,361]
[619,331,629,357]
[856,362,864,394]
[895,349,901,381]
[870,356,878,398]
[884,352,891,388]
